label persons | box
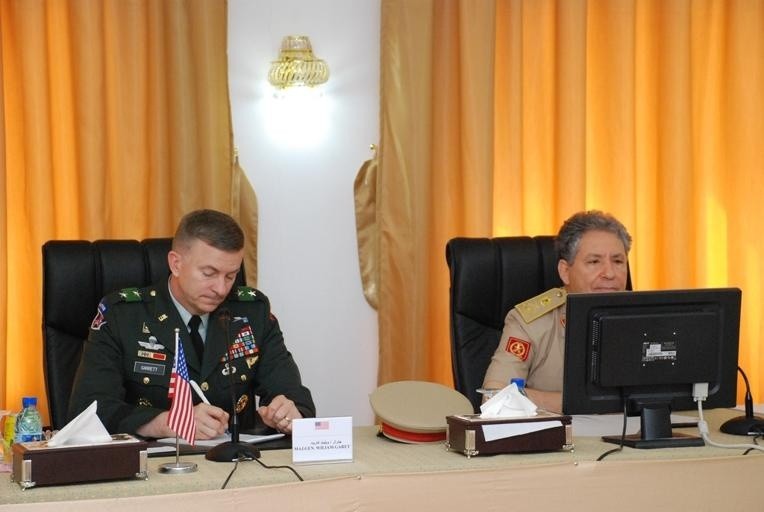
[481,208,631,418]
[63,208,316,444]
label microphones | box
[720,367,764,435]
[205,309,260,462]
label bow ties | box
[187,315,204,365]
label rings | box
[284,416,291,422]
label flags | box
[165,335,197,450]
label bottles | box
[15,397,43,442]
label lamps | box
[270,36,330,88]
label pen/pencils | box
[189,379,230,436]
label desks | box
[0,407,764,512]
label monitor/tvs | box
[562,288,741,448]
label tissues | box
[447,379,572,457]
[11,398,148,485]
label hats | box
[369,380,475,445]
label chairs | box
[445,236,633,412]
[43,239,247,433]
